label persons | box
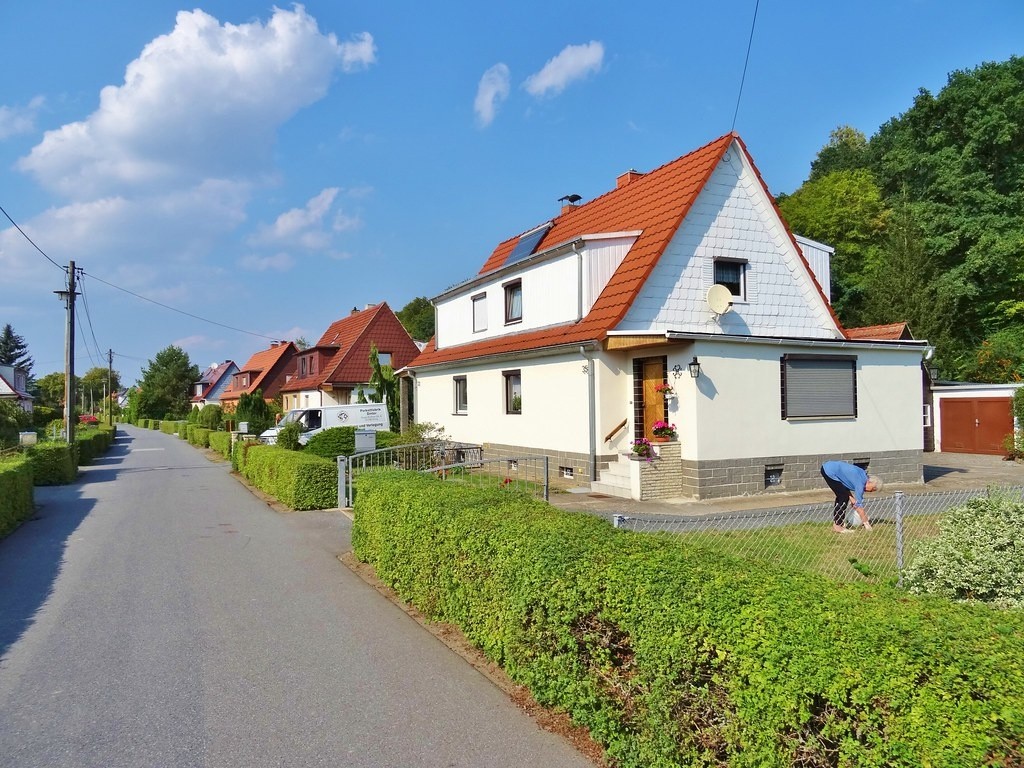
[122,413,124,417]
[276,413,282,425]
[820,461,883,535]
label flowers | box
[651,420,675,440]
[630,439,657,462]
[655,383,673,395]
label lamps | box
[927,367,939,380]
[688,357,700,378]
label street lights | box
[101,378,107,421]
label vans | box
[260,403,390,446]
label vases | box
[653,434,670,442]
[665,393,676,398]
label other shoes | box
[832,522,855,534]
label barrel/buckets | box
[848,507,869,527]
